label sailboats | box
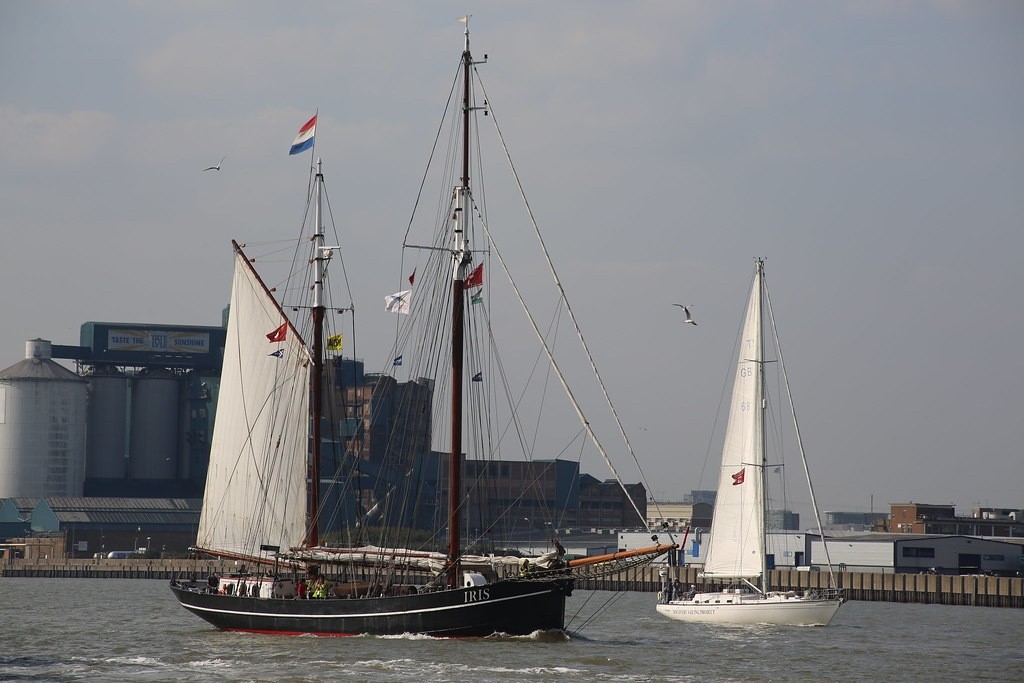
[656,256,844,630]
[169,13,681,638]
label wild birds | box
[202,155,227,173]
[672,302,698,326]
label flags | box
[732,467,745,485]
[289,115,317,155]
[471,288,483,305]
[266,322,287,342]
[267,349,284,359]
[327,334,342,350]
[472,372,482,381]
[409,268,416,285]
[332,355,342,367]
[463,263,483,289]
[385,289,412,314]
[393,355,402,365]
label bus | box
[108,551,135,559]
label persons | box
[664,578,697,603]
[519,559,533,577]
[298,575,333,599]
[207,572,219,594]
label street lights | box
[147,537,150,549]
[525,517,531,556]
